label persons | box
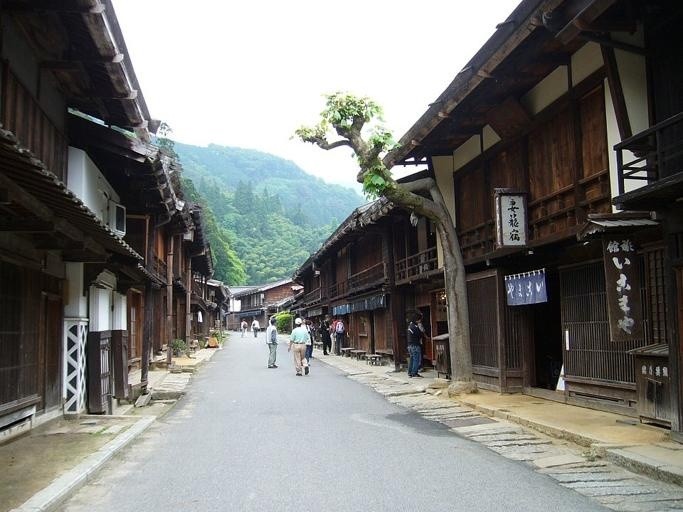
[241,318,248,338]
[288,318,309,376]
[267,319,278,368]
[251,319,259,338]
[415,312,430,372]
[301,314,345,365]
[407,313,423,378]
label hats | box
[295,317,302,325]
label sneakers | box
[324,352,329,355]
[268,363,278,368]
[296,373,302,375]
[305,367,309,375]
[408,368,426,379]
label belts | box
[293,342,304,344]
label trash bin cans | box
[434,334,451,373]
